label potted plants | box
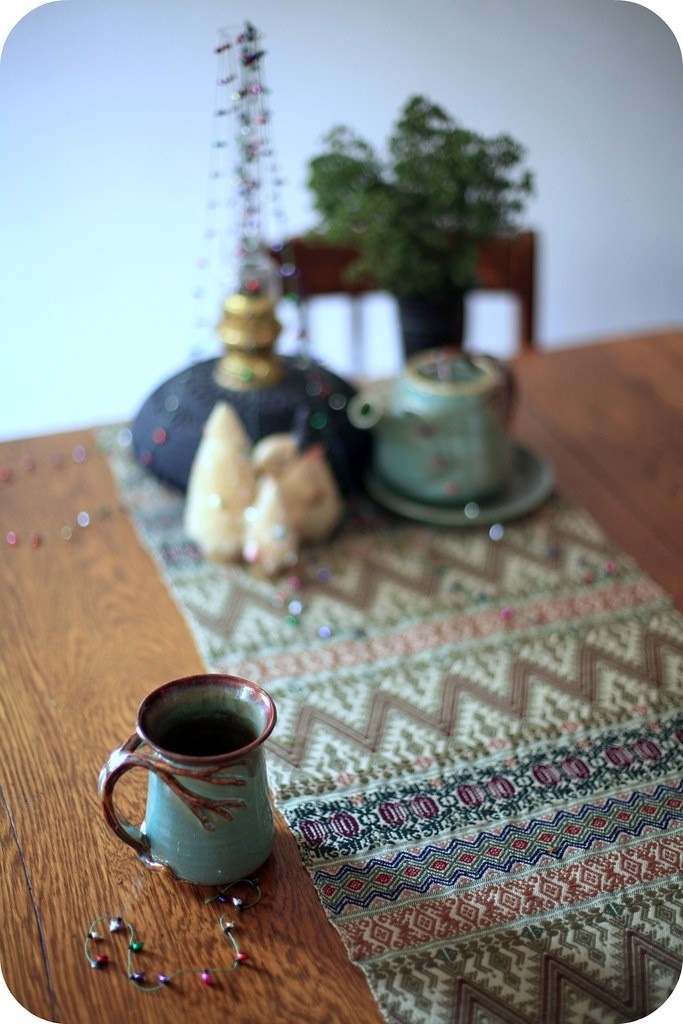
[307,95,535,362]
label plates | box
[364,437,556,527]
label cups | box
[98,673,278,886]
[364,348,517,507]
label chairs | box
[270,231,535,366]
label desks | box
[1,329,682,1022]
